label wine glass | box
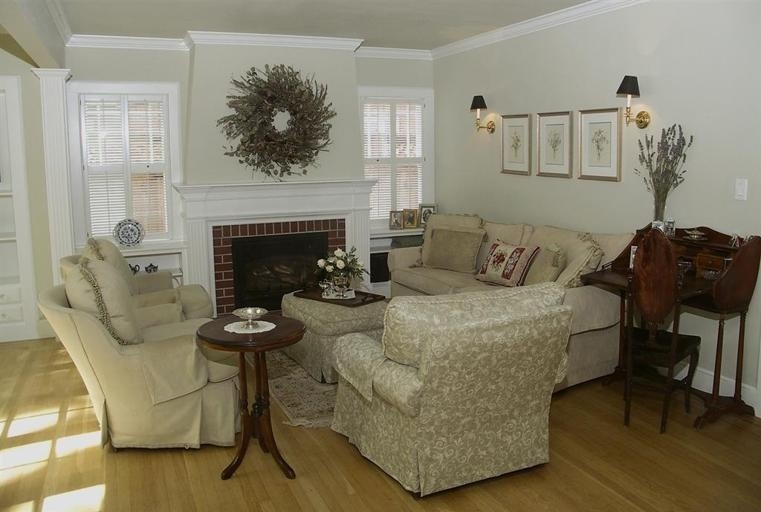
[234,305,268,330]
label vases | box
[332,276,351,299]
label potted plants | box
[632,122,696,233]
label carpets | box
[246,345,339,431]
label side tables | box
[195,308,308,482]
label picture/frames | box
[389,203,439,231]
[499,107,625,184]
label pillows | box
[420,227,568,289]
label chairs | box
[620,225,701,434]
[331,280,574,496]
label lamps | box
[469,92,496,137]
[614,74,653,129]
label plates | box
[112,219,146,247]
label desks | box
[578,220,761,430]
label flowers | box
[312,245,373,283]
[216,62,338,182]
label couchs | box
[387,211,640,400]
